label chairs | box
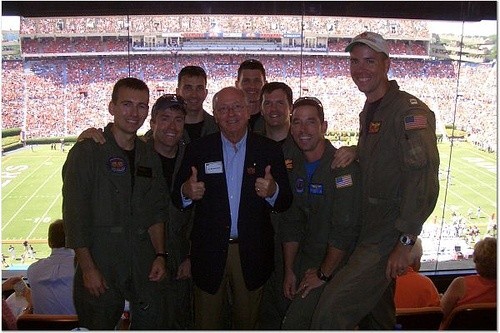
[396,306,442,330]
[16,314,79,330]
[445,302,497,330]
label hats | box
[345,32,389,57]
[152,94,187,115]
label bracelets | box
[24,306,33,313]
[155,251,169,261]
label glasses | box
[294,97,323,111]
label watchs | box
[317,268,332,281]
[399,234,415,246]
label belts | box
[229,238,239,244]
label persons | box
[76,57,360,331]
[2,240,37,268]
[2,274,34,330]
[1,14,498,155]
[437,236,497,330]
[313,32,440,331]
[418,162,497,262]
[172,86,295,331]
[393,237,439,308]
[26,219,78,316]
[61,76,169,332]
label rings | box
[258,187,260,191]
[303,283,307,288]
